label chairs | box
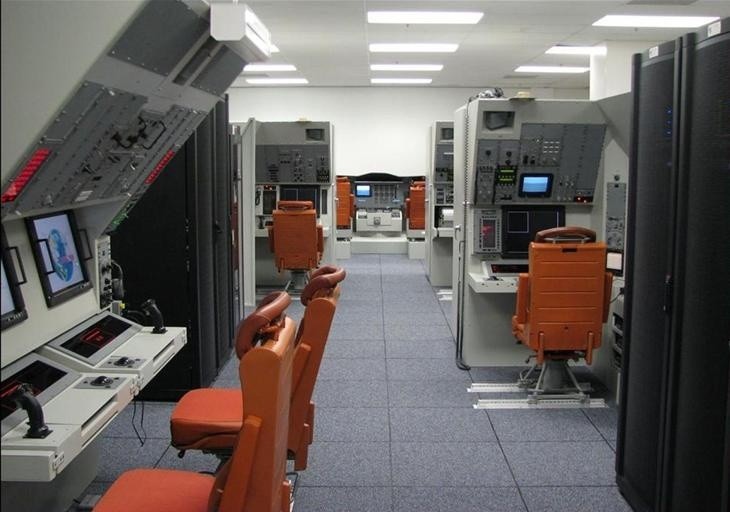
[267,201,324,295]
[335,177,355,241]
[171,266,346,503]
[511,226,614,404]
[91,291,297,511]
[405,181,426,241]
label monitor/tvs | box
[518,174,553,199]
[0,224,28,334]
[24,209,93,309]
[355,184,371,197]
